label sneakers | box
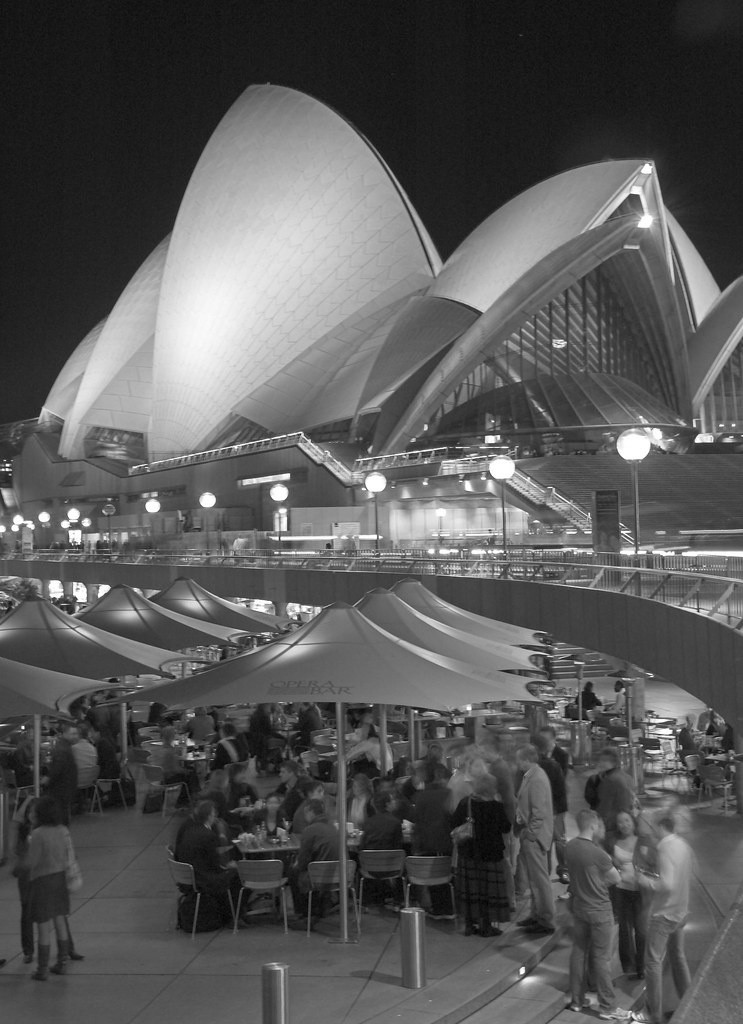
[599,1007,632,1021]
[570,997,592,1011]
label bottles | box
[256,825,262,843]
[261,822,266,840]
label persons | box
[307,543,333,570]
[566,812,694,1024]
[680,706,733,790]
[8,796,85,981]
[1,589,627,937]
[49,537,141,562]
[486,529,497,546]
[13,539,21,556]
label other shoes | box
[632,1011,659,1024]
[638,971,647,979]
[480,927,504,938]
[289,915,319,929]
[229,919,256,929]
[465,926,480,935]
[526,923,556,935]
[50,963,68,976]
[560,873,571,883]
[67,951,85,960]
[31,970,48,981]
[175,799,192,808]
[517,917,535,926]
[23,954,33,964]
[0,959,6,966]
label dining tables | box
[151,738,195,751]
[639,717,671,738]
[185,751,215,788]
[232,832,410,908]
[387,713,439,740]
[705,753,740,795]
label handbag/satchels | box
[178,893,224,932]
[449,794,475,848]
[111,777,136,806]
[63,824,83,893]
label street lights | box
[68,508,80,550]
[144,498,161,564]
[269,483,290,568]
[488,453,517,579]
[363,469,387,565]
[102,503,116,564]
[38,512,51,551]
[617,429,651,595]
[199,492,215,564]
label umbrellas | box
[96,601,555,939]
[0,656,144,723]
[389,579,546,758]
[72,584,261,762]
[193,587,552,780]
[1,592,212,799]
[150,577,305,677]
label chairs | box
[487,681,739,817]
[1,702,483,939]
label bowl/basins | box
[268,836,280,844]
